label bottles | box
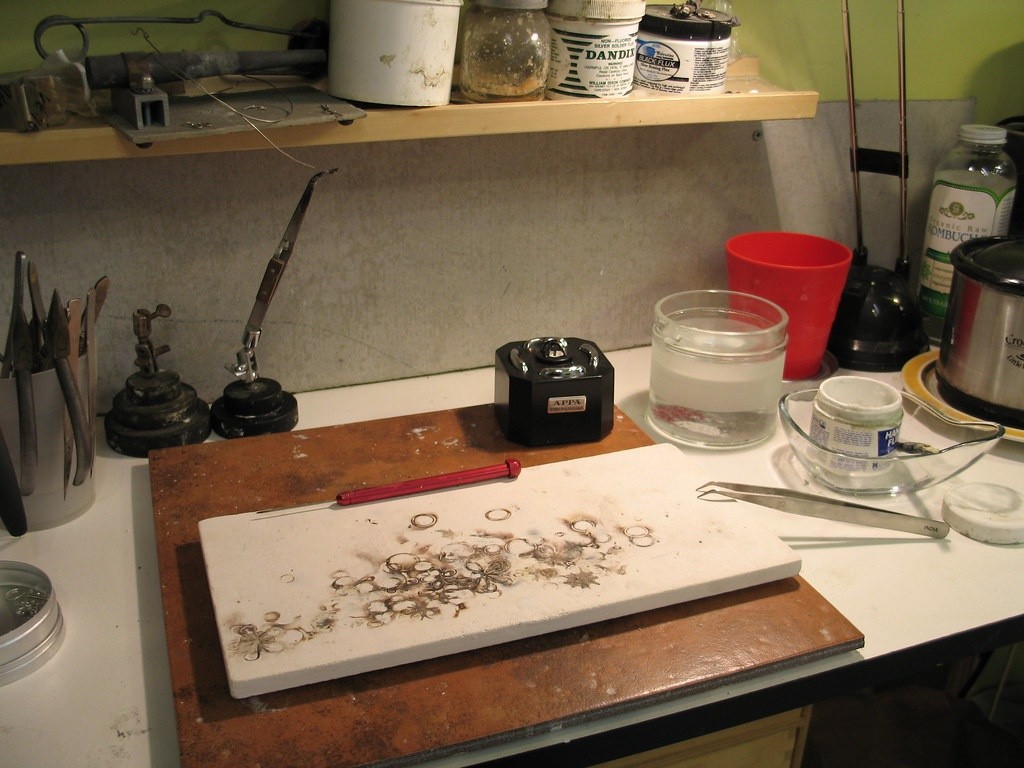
[920,124,1017,344]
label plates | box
[900,351,1024,444]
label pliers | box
[27,259,97,486]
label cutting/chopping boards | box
[149,404,868,768]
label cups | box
[725,231,852,380]
[328,0,464,105]
[648,288,788,449]
[1,345,97,528]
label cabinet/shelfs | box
[585,703,814,768]
